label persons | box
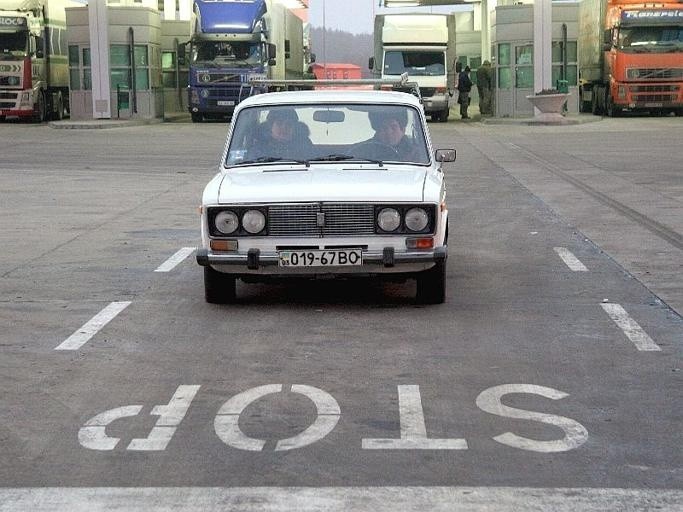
[353,110,427,162]
[476,59,493,114]
[458,66,474,118]
[247,108,312,160]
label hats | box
[465,66,470,72]
[483,60,490,65]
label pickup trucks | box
[197,73,457,306]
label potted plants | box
[527,89,571,113]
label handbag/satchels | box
[458,96,471,105]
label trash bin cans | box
[556,79,568,116]
[116,83,128,121]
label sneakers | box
[462,117,471,119]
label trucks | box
[578,1,683,117]
[177,0,304,123]
[0,0,86,124]
[368,14,463,122]
